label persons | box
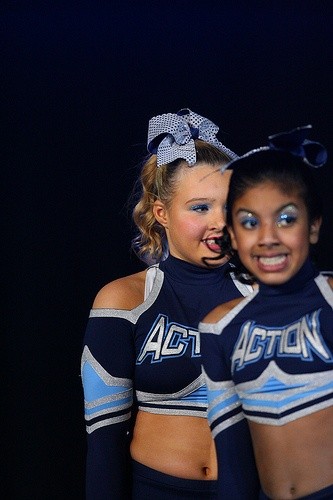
[77,103,241,500]
[201,128,332,500]
